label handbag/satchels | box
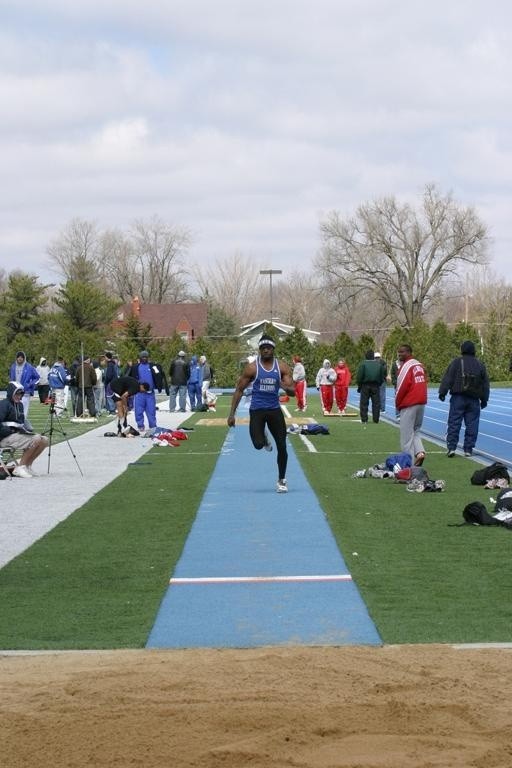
[471,462,510,485]
[460,370,484,395]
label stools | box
[0,448,18,476]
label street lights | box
[259,269,283,323]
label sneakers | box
[275,479,288,494]
[414,452,424,466]
[446,449,455,457]
[465,452,473,456]
[12,465,39,478]
[294,404,346,416]
[263,430,272,452]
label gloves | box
[439,391,445,401]
[481,399,487,409]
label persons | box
[292,353,308,413]
[438,340,490,457]
[0,349,212,478]
[316,357,352,415]
[227,334,296,493]
[355,344,427,466]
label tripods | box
[41,413,83,477]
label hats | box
[58,350,206,363]
